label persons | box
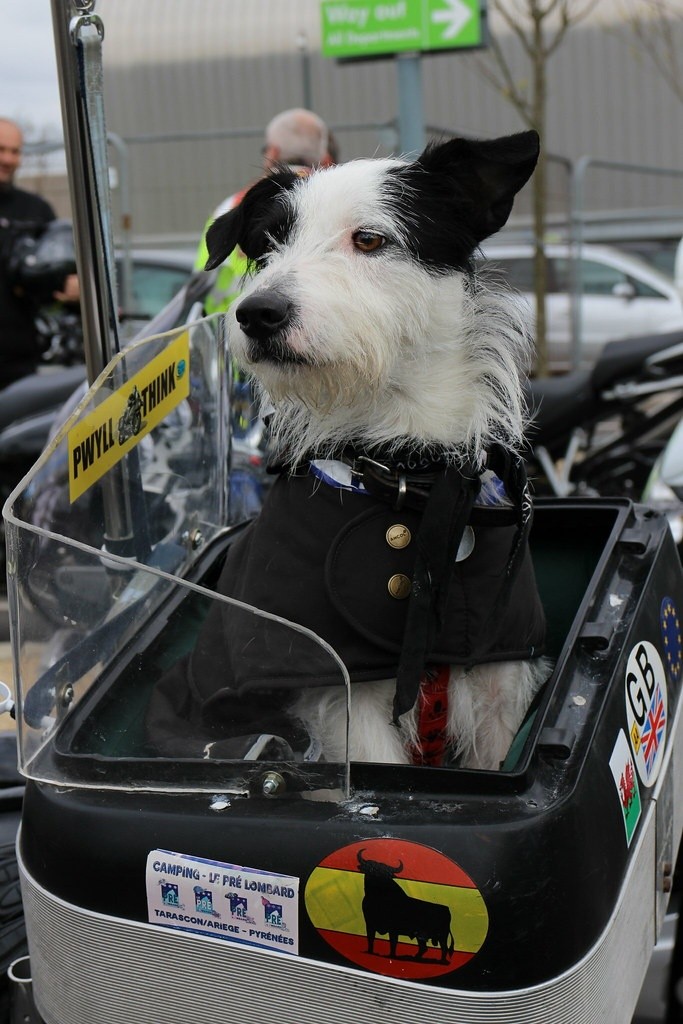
[195,108,330,429]
[0,117,80,611]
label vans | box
[469,248,681,375]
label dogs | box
[205,129,542,771]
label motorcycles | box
[0,269,683,630]
